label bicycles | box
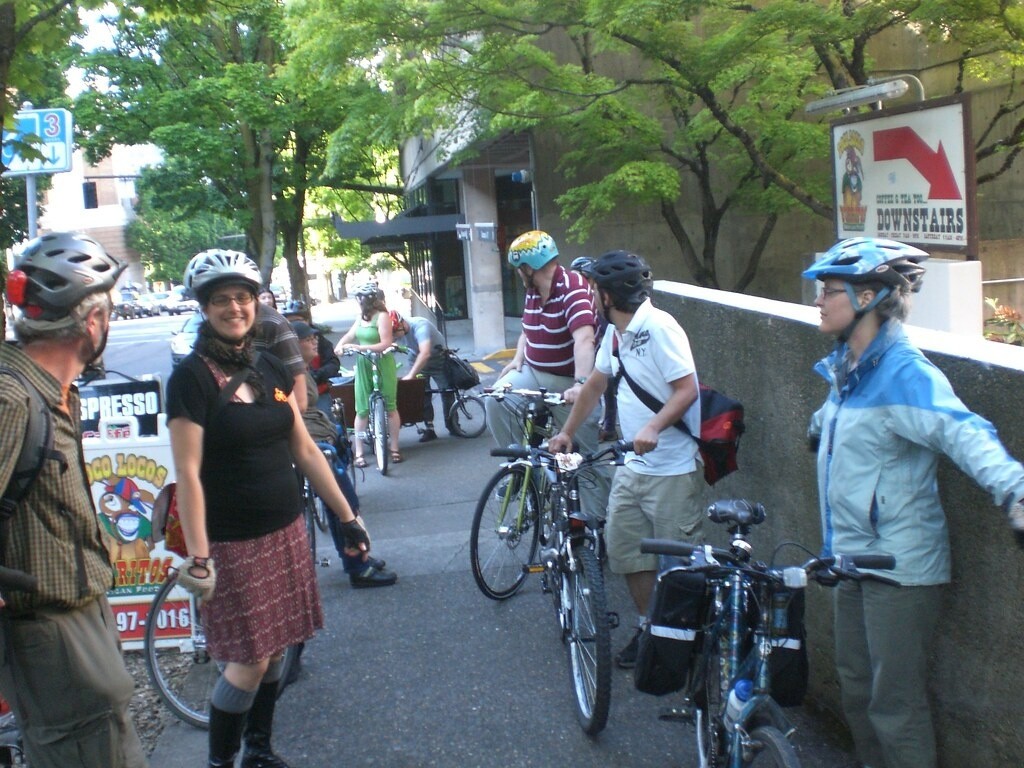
[327,345,488,440]
[301,398,357,533]
[343,343,411,477]
[638,497,901,768]
[489,439,649,737]
[470,381,568,602]
[144,480,332,732]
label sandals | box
[355,454,368,467]
[391,450,400,463]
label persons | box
[375,281,386,303]
[336,279,400,466]
[802,237,1024,768]
[548,250,703,666]
[179,250,398,588]
[166,251,371,768]
[390,309,466,441]
[0,232,151,768]
[485,230,617,591]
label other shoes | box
[589,536,606,563]
[419,429,437,442]
[615,623,645,667]
[343,555,397,589]
[495,475,523,503]
[450,424,466,436]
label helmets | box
[589,250,652,301]
[507,229,558,268]
[802,236,930,293]
[7,232,128,322]
[277,300,309,319]
[182,248,263,302]
[390,310,403,332]
[571,255,593,271]
[347,279,378,295]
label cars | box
[109,283,289,322]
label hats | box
[290,321,319,338]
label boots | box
[240,680,291,768]
[207,702,247,768]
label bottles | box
[723,680,754,733]
[773,593,792,638]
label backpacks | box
[448,351,479,390]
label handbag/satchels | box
[151,483,189,561]
[699,382,746,486]
[635,566,809,712]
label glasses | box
[206,294,253,307]
[820,287,847,298]
[300,336,319,341]
[586,283,611,295]
